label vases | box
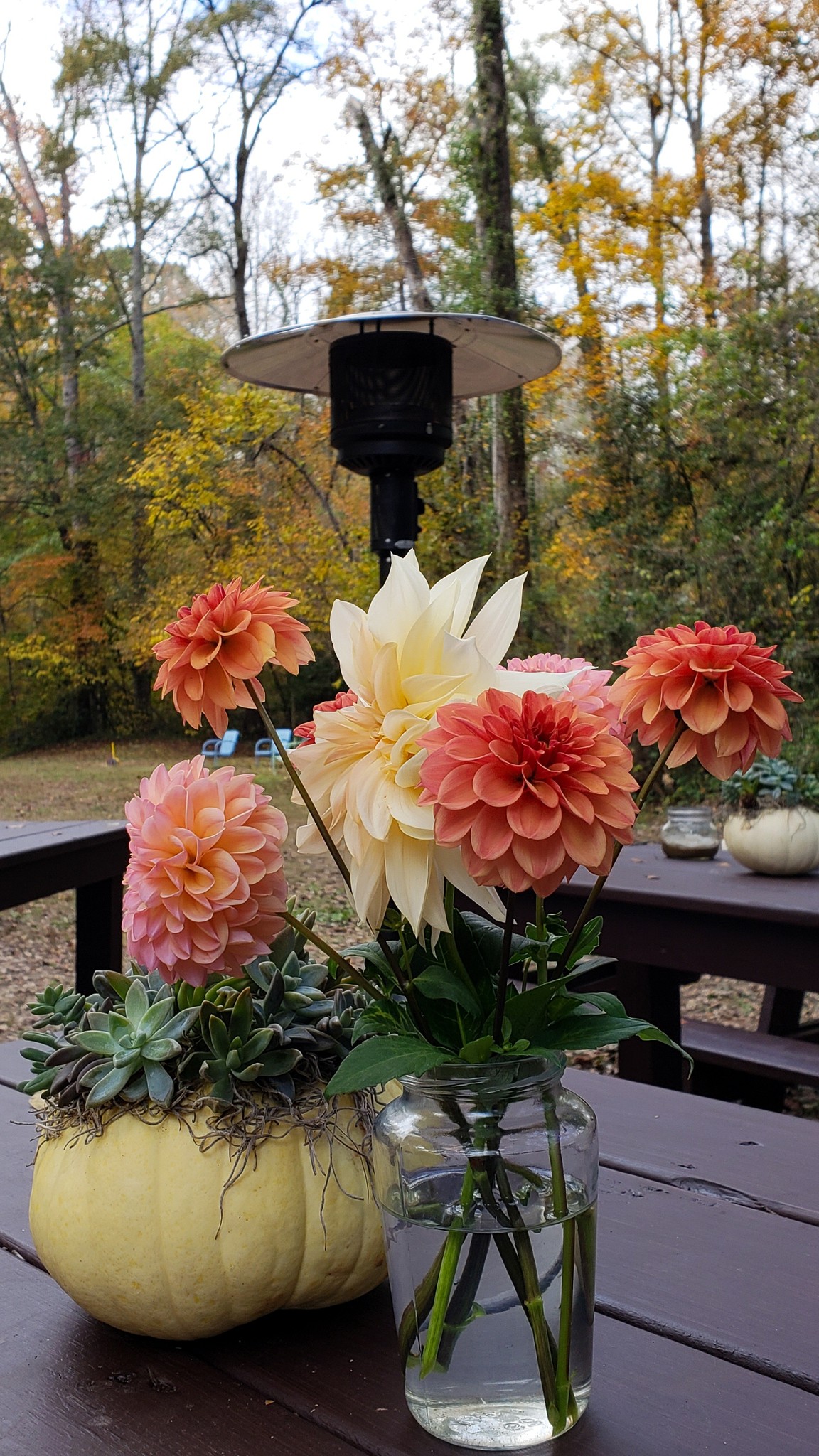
[365,1059,597,1454]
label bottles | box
[661,807,719,858]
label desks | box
[0,1020,818,1456]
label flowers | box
[106,550,810,1440]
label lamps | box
[215,310,560,590]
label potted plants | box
[719,756,818,872]
[6,898,444,1338]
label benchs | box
[0,820,127,1005]
[516,847,817,1121]
